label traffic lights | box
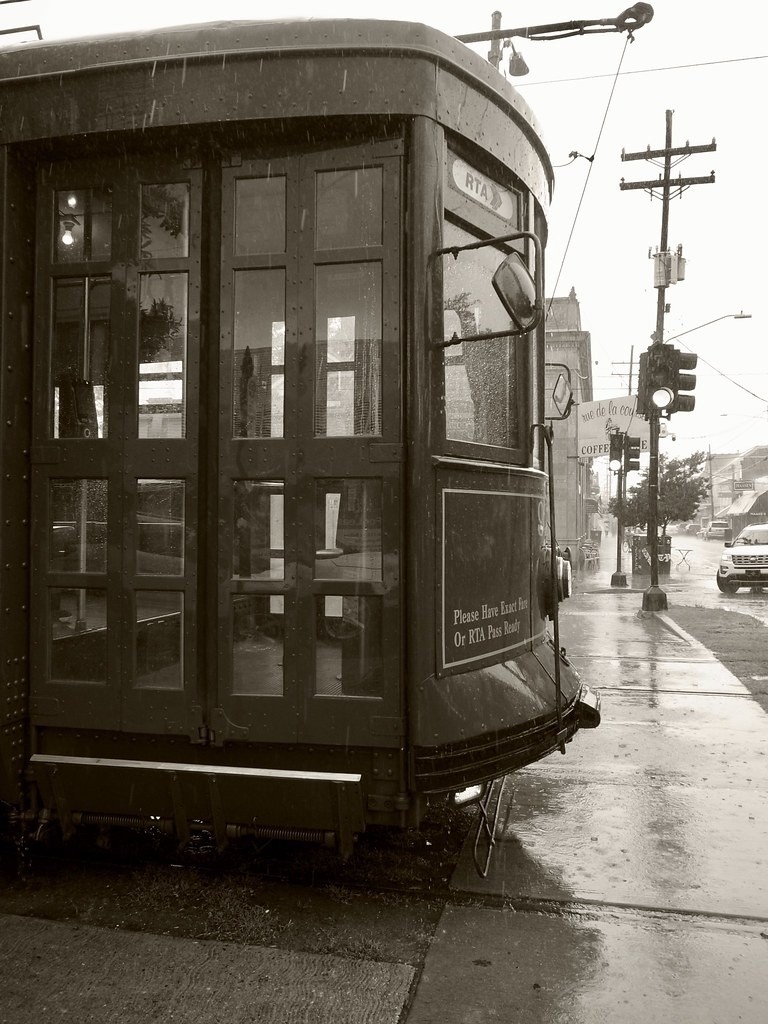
[674,348,697,413]
[608,434,622,471]
[624,435,640,473]
[645,343,677,414]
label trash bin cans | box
[633,533,672,575]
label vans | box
[678,523,688,534]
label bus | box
[0,17,598,883]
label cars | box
[696,528,705,538]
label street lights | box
[641,312,753,611]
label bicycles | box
[622,533,633,553]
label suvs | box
[685,525,701,536]
[704,521,730,541]
[716,523,768,594]
